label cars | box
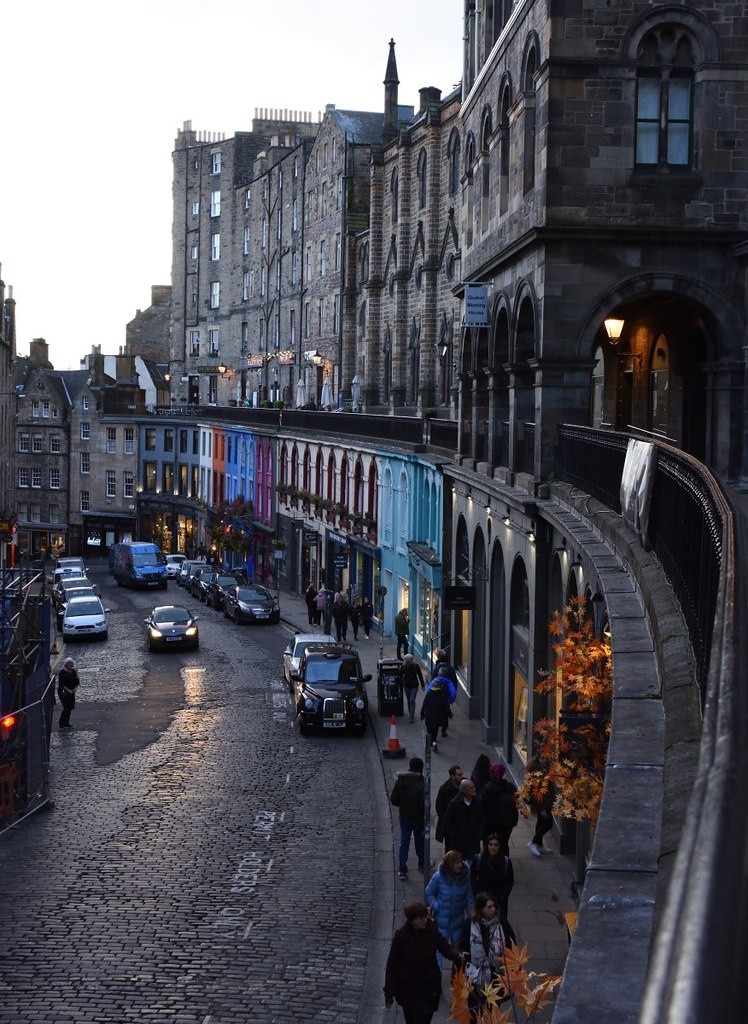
[283,634,339,693]
[165,554,189,577]
[50,556,98,611]
[175,559,280,625]
[143,604,201,653]
[58,595,110,644]
[54,586,103,627]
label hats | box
[404,654,413,662]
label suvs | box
[290,641,373,735]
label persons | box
[349,595,375,641]
[395,608,410,661]
[526,757,558,857]
[399,654,425,723]
[420,649,458,753]
[305,583,327,627]
[57,657,81,728]
[195,541,217,565]
[331,589,349,642]
[383,754,518,1024]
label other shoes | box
[60,723,64,728]
[313,624,321,628]
[337,634,369,642]
[66,724,73,727]
[409,717,414,723]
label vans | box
[109,542,168,591]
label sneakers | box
[399,871,407,879]
[433,742,438,753]
[418,860,436,872]
[539,847,553,854]
[526,840,541,856]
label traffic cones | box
[381,713,407,758]
[50,638,60,655]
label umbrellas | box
[350,374,361,414]
[295,379,309,410]
[320,376,334,411]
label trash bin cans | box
[377,659,404,718]
